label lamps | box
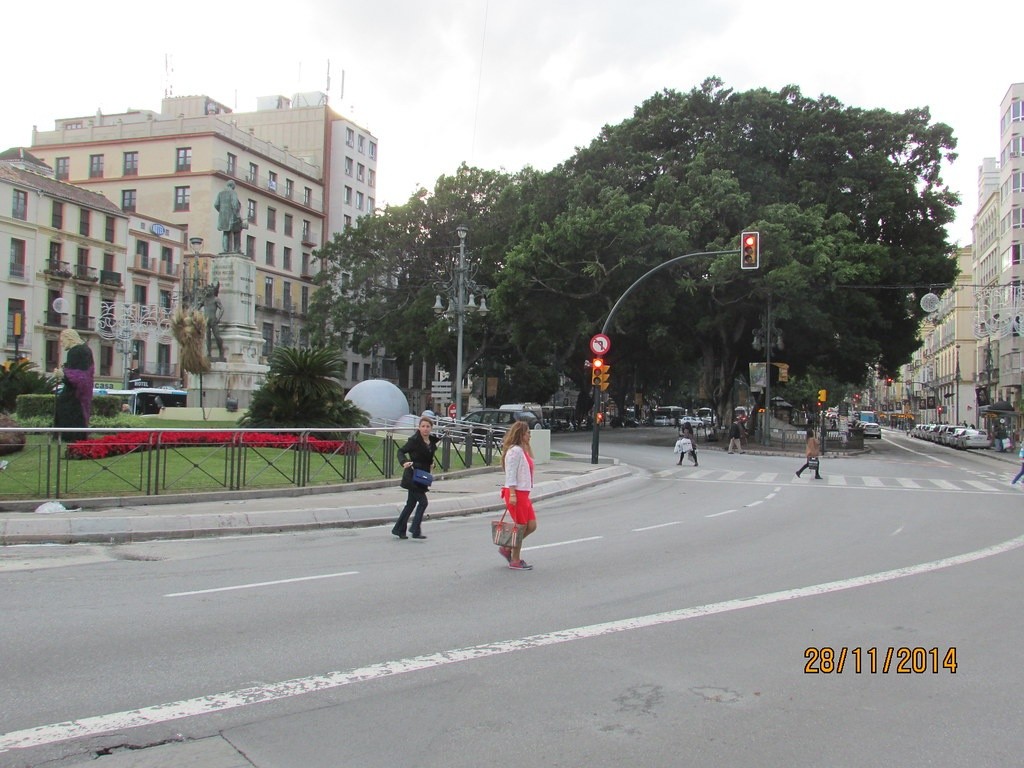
[53,298,68,313]
[921,288,941,312]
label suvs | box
[458,409,545,450]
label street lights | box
[123,303,130,389]
[184,237,207,407]
[431,225,489,438]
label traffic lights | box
[591,358,610,393]
[737,231,759,271]
[759,409,765,413]
[937,407,942,414]
[852,394,861,404]
[818,389,826,403]
[887,378,892,386]
[816,402,823,407]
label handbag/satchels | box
[673,438,693,454]
[491,503,526,547]
[412,468,433,487]
[808,457,818,470]
[687,443,698,463]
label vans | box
[859,411,878,424]
[499,403,543,432]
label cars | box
[910,424,993,451]
[652,416,669,426]
[855,420,882,439]
[678,417,704,427]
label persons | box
[214,180,244,254]
[727,418,745,454]
[1011,437,1024,484]
[796,428,822,479]
[55,328,94,442]
[198,281,227,362]
[392,418,441,539]
[932,421,975,429]
[499,421,537,570]
[996,426,1007,453]
[676,422,698,466]
[830,418,836,430]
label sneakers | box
[508,560,533,570]
[498,546,511,562]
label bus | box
[89,386,187,417]
[540,405,587,430]
[656,405,684,425]
[695,407,717,425]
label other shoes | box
[815,476,823,479]
[413,533,426,539]
[694,462,698,466]
[796,471,800,478]
[391,531,408,539]
[676,461,682,465]
